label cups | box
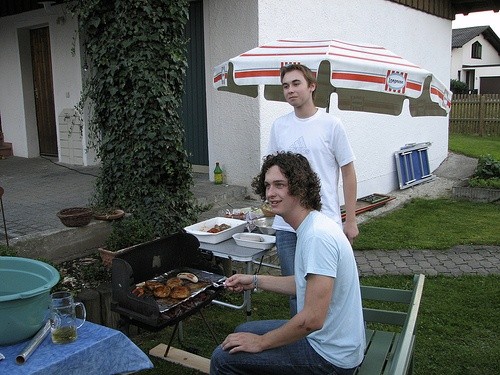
[49,292,86,345]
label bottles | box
[214,162,223,184]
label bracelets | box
[253,275,257,289]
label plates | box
[95,209,125,220]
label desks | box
[0,310,154,375]
[199,227,281,322]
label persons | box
[210,151,366,375]
[269,64,359,318]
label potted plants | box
[55,0,214,269]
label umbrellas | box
[214,39,453,118]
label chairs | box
[353,273,426,375]
[394,141,433,190]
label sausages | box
[176,272,198,283]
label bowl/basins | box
[232,232,276,250]
[0,256,60,346]
[183,216,248,244]
[253,216,277,235]
[57,207,95,227]
